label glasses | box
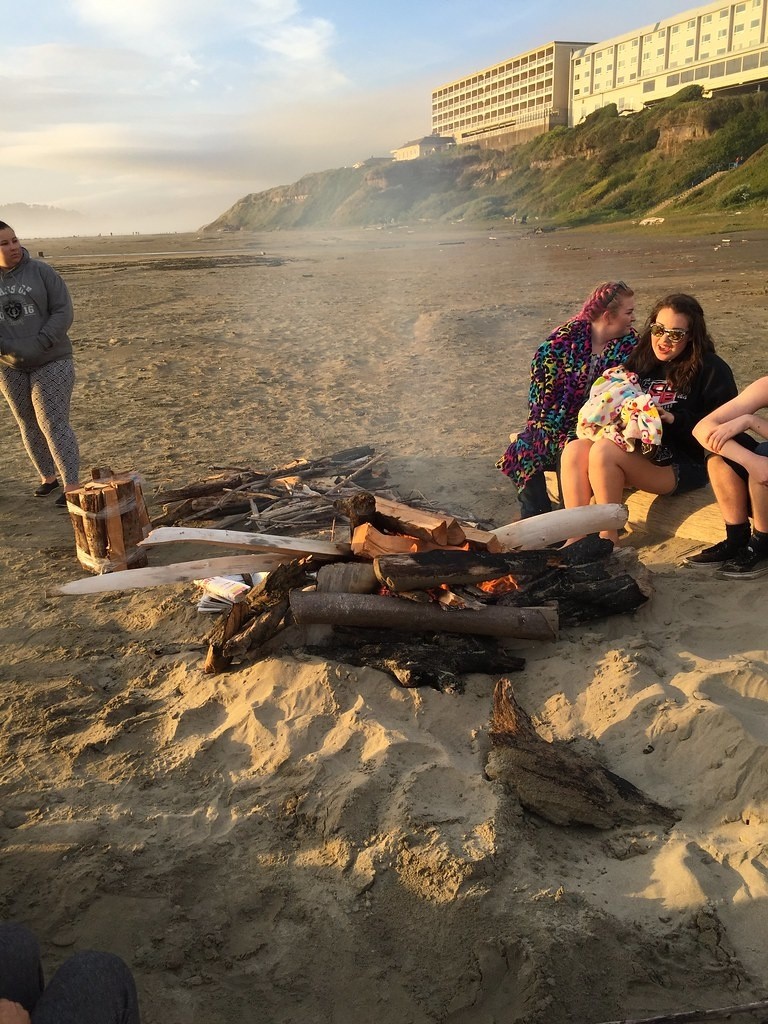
[650,322,691,342]
[605,280,626,308]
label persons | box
[559,292,738,554]
[682,375,768,581]
[0,221,78,506]
[495,280,642,520]
[0,922,140,1024]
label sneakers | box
[683,540,749,568]
[712,542,768,579]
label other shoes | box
[55,491,68,507]
[34,479,60,497]
